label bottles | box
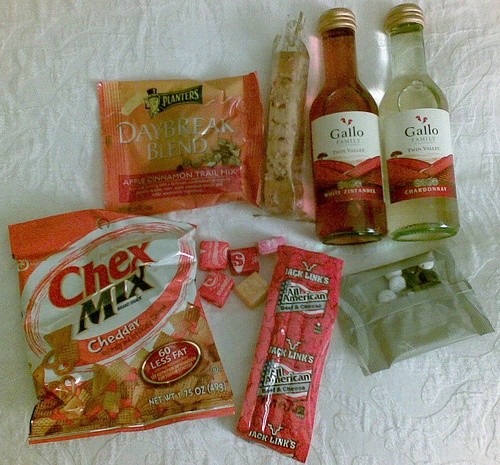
[377,4,463,241]
[310,7,389,245]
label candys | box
[262,51,311,217]
[376,259,440,304]
